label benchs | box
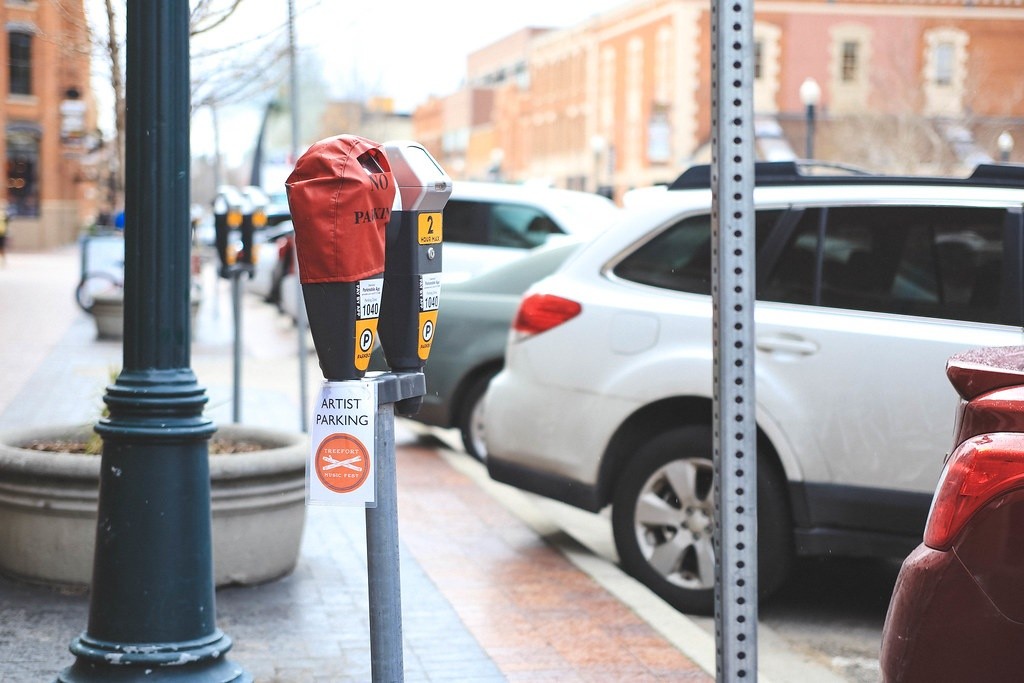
[769,247,936,313]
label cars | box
[366,180,621,465]
[878,343,1024,683]
[189,189,313,328]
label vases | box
[90,293,201,340]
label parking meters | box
[283,131,454,683]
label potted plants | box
[0,368,309,587]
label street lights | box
[798,76,822,164]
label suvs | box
[483,159,1024,618]
